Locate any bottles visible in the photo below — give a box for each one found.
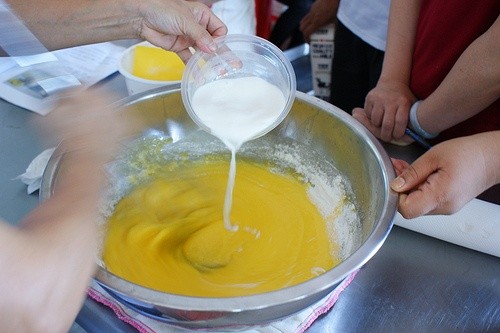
[310,24,335,97]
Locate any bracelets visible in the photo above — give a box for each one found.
[406,100,436,145]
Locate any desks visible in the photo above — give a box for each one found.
[0,61,500,333]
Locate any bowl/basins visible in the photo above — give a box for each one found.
[39,82,400,329]
[116,41,182,96]
[181,33,297,142]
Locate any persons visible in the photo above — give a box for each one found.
[298,0,500,219]
[0,1,245,333]
